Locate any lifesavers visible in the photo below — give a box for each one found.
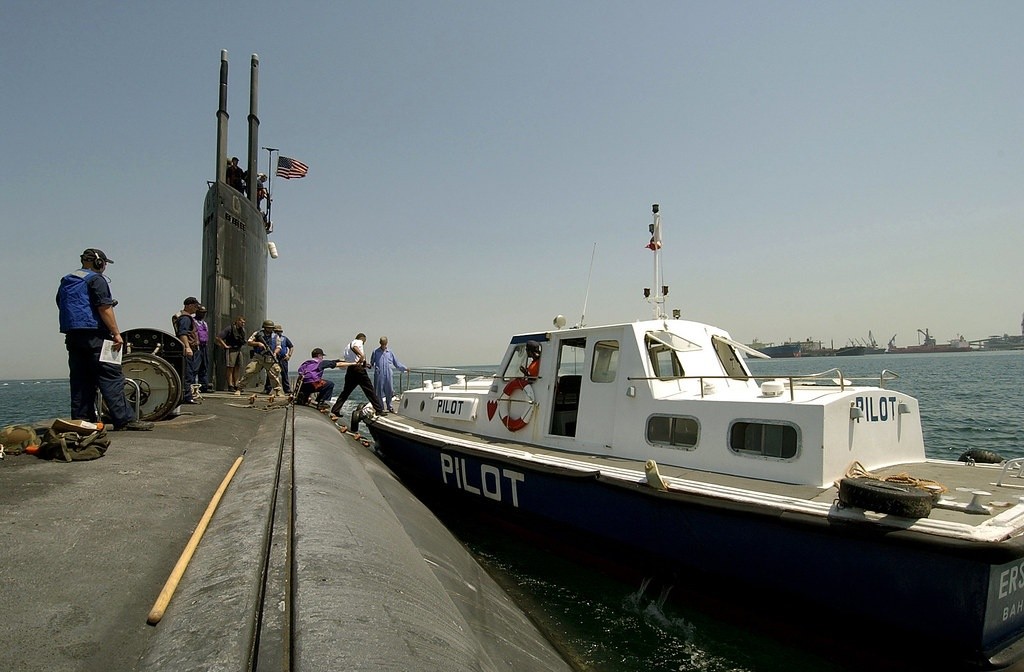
[838,475,933,519]
[498,377,536,433]
[351,410,359,431]
[958,446,1005,464]
[356,401,367,420]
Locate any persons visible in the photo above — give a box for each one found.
[296,349,361,409]
[331,333,388,416]
[258,324,294,396]
[519,341,540,377]
[234,320,284,398]
[227,157,247,195]
[193,305,214,392]
[367,336,408,413]
[244,171,263,184]
[257,175,268,211]
[175,297,201,403]
[56,248,154,429]
[214,316,246,393]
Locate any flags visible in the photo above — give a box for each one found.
[276,156,308,179]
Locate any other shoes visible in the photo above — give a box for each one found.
[317,400,331,410]
[202,388,214,393]
[376,409,387,416]
[184,399,200,405]
[390,407,397,414]
[228,386,236,392]
[285,389,293,394]
[333,411,344,417]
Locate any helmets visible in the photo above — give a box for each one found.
[198,305,206,312]
[262,320,275,328]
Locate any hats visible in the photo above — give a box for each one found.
[273,325,283,331]
[312,349,326,357]
[84,248,113,263]
[527,341,541,359]
[184,296,201,305]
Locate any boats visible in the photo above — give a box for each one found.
[355,202,1024,672]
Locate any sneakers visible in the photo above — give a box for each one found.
[114,418,154,432]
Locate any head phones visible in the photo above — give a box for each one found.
[88,249,104,270]
